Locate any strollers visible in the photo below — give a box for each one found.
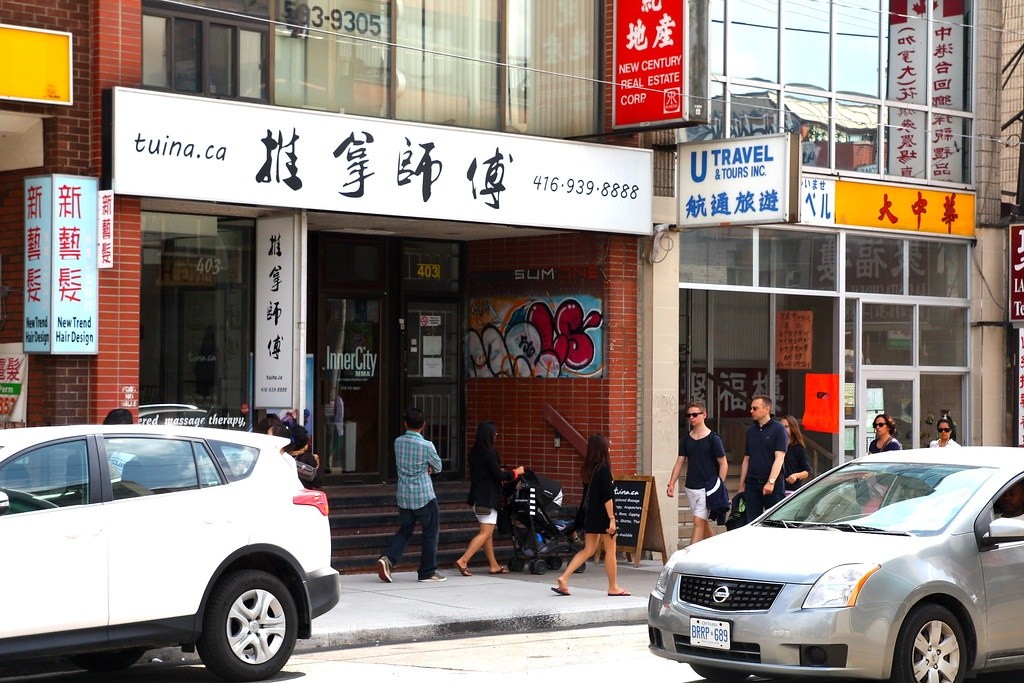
[499,463,587,575]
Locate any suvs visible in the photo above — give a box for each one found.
[0,423,341,683]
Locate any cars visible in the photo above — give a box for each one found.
[645,445,1023,683]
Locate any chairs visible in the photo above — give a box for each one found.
[56,456,84,504]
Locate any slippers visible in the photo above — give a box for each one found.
[608,590,631,596]
[551,587,571,595]
[455,561,472,575]
[490,565,510,574]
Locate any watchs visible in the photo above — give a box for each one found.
[768,479,774,483]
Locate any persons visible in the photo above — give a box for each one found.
[666,401,728,546]
[738,396,789,524]
[101,408,322,515]
[779,415,813,492]
[556,434,632,596]
[377,408,447,583]
[859,414,903,520]
[930,415,961,448]
[455,421,525,576]
[326,386,345,465]
[993,482,1024,520]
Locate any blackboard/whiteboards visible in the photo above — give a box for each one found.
[595,475,668,552]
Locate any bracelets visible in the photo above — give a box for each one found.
[667,483,674,488]
[610,517,615,520]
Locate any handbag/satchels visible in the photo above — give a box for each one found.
[295,460,316,480]
[475,501,492,515]
[573,494,589,529]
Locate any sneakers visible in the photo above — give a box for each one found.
[377,556,393,582]
[419,572,446,582]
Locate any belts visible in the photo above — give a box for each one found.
[752,479,766,484]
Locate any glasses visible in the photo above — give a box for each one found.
[873,422,888,428]
[686,412,704,418]
[937,427,950,433]
[749,406,766,410]
